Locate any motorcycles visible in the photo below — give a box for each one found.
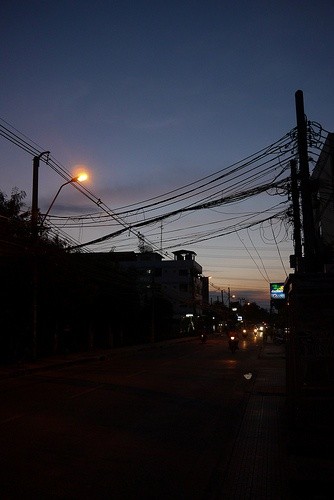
[200,331,208,345]
[227,335,239,353]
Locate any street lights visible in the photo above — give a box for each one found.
[38,178,79,239]
